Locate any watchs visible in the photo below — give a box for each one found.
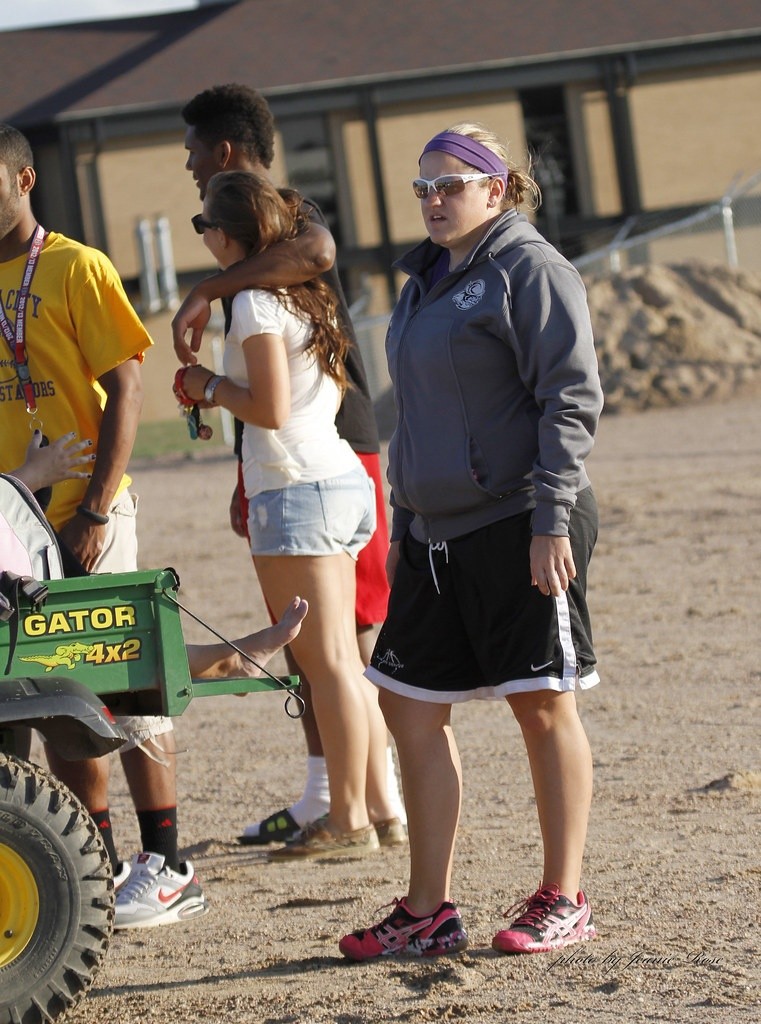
[205,376,227,405]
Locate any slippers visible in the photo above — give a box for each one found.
[237,808,300,844]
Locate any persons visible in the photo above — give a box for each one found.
[0,84,408,930]
[339,122,607,959]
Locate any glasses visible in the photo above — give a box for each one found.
[191,214,229,234]
[412,172,505,198]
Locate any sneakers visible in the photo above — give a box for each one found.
[113,852,210,930]
[339,895,469,961]
[375,817,406,845]
[491,880,596,954]
[114,860,133,889]
[267,814,380,863]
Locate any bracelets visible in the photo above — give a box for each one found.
[76,505,109,524]
[203,373,215,394]
